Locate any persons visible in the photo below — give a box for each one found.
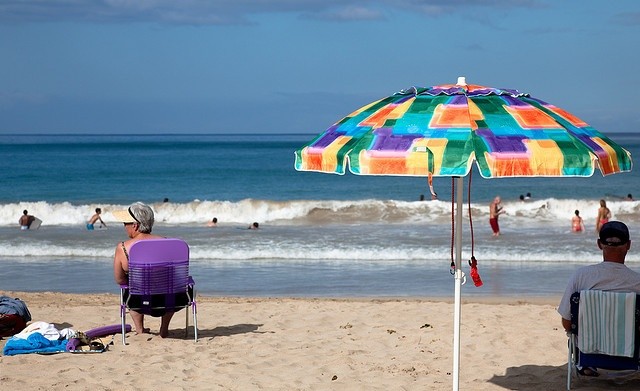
[519,194,524,204]
[19,210,33,229]
[572,210,582,233]
[490,196,504,236]
[595,200,610,232]
[108,201,172,337]
[524,193,531,199]
[87,208,107,230]
[164,198,168,202]
[557,221,640,332]
[253,222,258,230]
[625,193,633,201]
[419,194,425,200]
[208,218,217,227]
[432,192,437,201]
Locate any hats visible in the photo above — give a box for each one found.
[112,206,140,224]
[599,221,630,245]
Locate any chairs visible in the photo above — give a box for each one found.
[119,239,197,346]
[566,289,640,391]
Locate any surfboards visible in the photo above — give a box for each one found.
[94,227,108,230]
[19,216,42,229]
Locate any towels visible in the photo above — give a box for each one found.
[576,290,636,357]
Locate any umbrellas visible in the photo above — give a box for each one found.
[295,77,633,391]
